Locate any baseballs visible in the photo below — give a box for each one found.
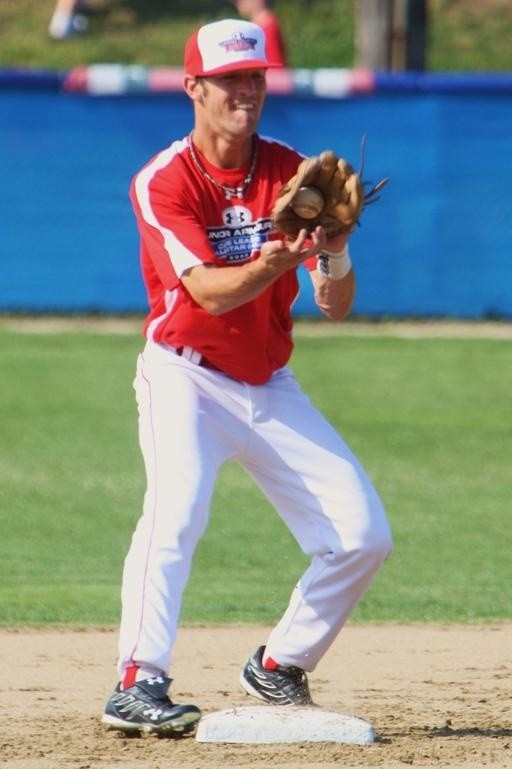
[293,184,323,218]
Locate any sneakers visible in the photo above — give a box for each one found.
[100,674,202,734]
[240,645,313,708]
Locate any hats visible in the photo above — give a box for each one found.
[184,18,285,78]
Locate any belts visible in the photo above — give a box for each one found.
[176,344,225,373]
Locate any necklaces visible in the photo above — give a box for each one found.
[184,129,262,195]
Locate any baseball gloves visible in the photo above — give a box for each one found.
[270,148,389,241]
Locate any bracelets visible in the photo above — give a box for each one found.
[316,239,352,281]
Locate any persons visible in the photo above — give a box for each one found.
[91,11,400,737]
[234,0,288,71]
